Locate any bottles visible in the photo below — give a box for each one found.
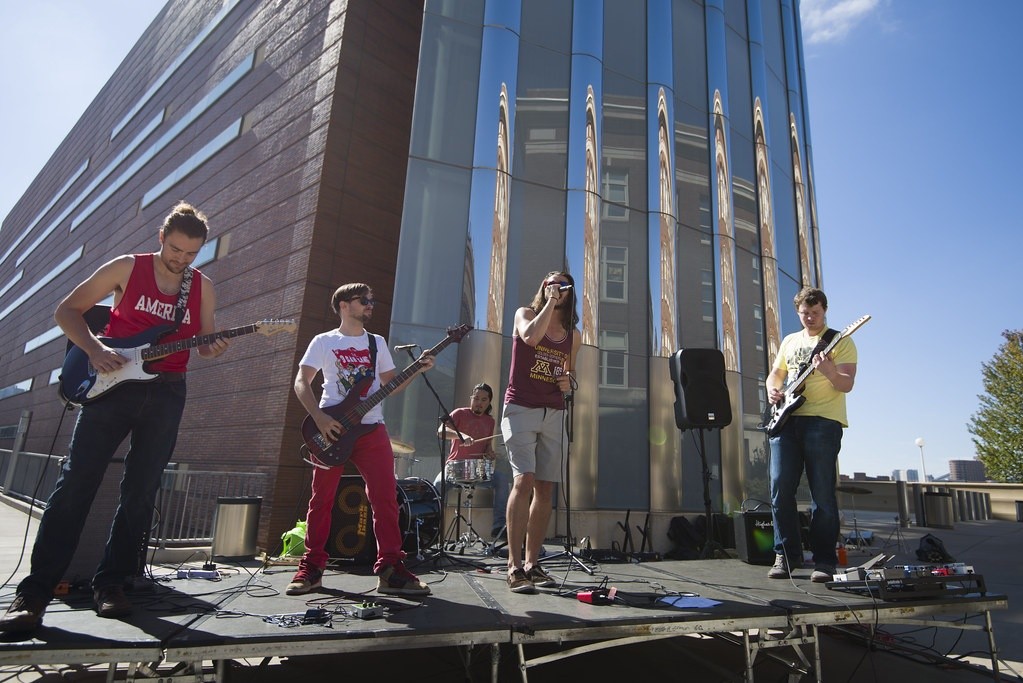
[839,544,848,566]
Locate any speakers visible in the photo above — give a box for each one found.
[668,348,732,431]
[60,430,157,587]
[733,510,805,566]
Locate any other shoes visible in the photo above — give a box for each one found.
[491,527,508,540]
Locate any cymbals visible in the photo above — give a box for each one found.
[836,486,874,496]
[496,442,506,447]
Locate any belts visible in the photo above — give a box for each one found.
[154,372,185,381]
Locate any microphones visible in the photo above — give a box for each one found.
[394,344,418,353]
[558,285,572,293]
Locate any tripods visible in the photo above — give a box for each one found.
[844,492,913,556]
[537,389,596,575]
[405,350,492,574]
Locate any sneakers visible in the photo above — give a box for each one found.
[768,554,801,578]
[507,568,535,592]
[525,566,556,585]
[378,562,430,593]
[0,596,42,630]
[286,559,321,594]
[94,585,134,617]
[811,562,835,581]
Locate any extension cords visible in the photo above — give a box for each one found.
[177,570,217,579]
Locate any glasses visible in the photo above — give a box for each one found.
[548,282,571,287]
[342,296,376,306]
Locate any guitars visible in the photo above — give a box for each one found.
[60,309,298,407]
[300,322,476,467]
[761,313,872,438]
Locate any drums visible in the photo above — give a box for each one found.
[445,457,496,485]
[395,478,444,554]
[390,439,419,480]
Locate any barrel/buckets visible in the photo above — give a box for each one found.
[211,495,262,562]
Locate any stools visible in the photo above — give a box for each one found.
[448,483,489,556]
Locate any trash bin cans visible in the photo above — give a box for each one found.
[210,495,263,562]
[1015,500,1023,522]
[924,492,955,528]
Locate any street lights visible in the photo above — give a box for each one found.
[915,437,927,482]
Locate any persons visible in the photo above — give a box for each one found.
[285,284,436,595]
[434,383,508,541]
[0,204,232,630]
[766,288,859,583]
[501,271,582,594]
